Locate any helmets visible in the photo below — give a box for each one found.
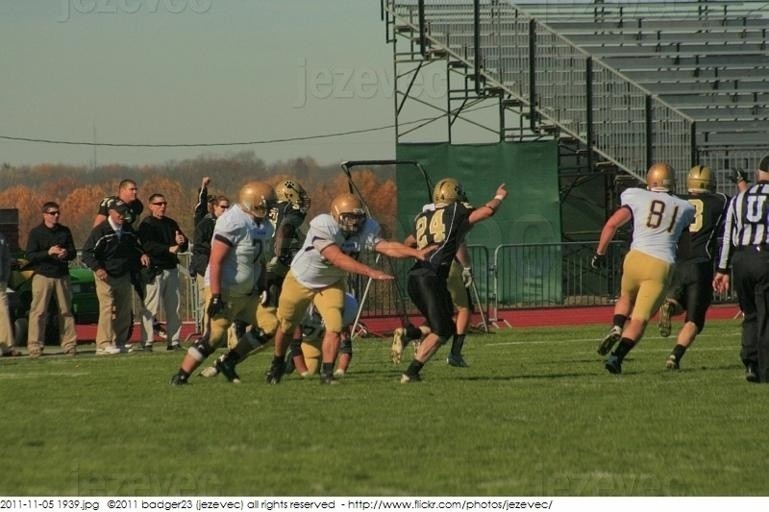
[433,177,464,205]
[647,163,715,193]
[330,194,367,235]
[240,180,310,220]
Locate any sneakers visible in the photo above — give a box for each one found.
[266,350,347,389]
[667,355,680,369]
[169,354,241,384]
[658,303,671,337]
[392,327,469,384]
[598,325,623,374]
[95,344,185,355]
[29,349,42,359]
[4,349,22,356]
[66,347,76,356]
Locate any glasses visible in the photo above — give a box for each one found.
[153,202,166,206]
[47,211,60,215]
[220,205,229,208]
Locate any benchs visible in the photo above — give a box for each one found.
[394,0,769,196]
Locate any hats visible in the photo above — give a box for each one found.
[107,198,128,212]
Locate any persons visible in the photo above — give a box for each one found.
[591,163,696,374]
[0,235,21,356]
[81,198,151,356]
[172,177,507,386]
[137,194,188,352]
[713,157,769,383]
[25,202,76,358]
[92,179,168,349]
[659,163,748,369]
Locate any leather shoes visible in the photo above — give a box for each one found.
[746,364,759,382]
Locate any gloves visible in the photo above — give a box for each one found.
[591,254,606,270]
[725,166,746,183]
[207,293,223,316]
[258,287,269,306]
[461,267,472,287]
